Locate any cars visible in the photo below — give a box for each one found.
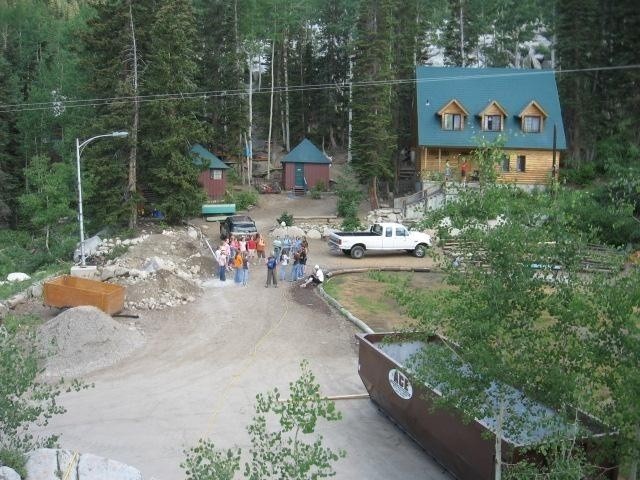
[219,214,258,240]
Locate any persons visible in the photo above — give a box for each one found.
[213,233,309,288]
[460,158,468,185]
[302,264,326,286]
[445,160,454,180]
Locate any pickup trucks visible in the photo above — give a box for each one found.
[330,222,431,258]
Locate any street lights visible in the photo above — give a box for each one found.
[75,131,129,267]
